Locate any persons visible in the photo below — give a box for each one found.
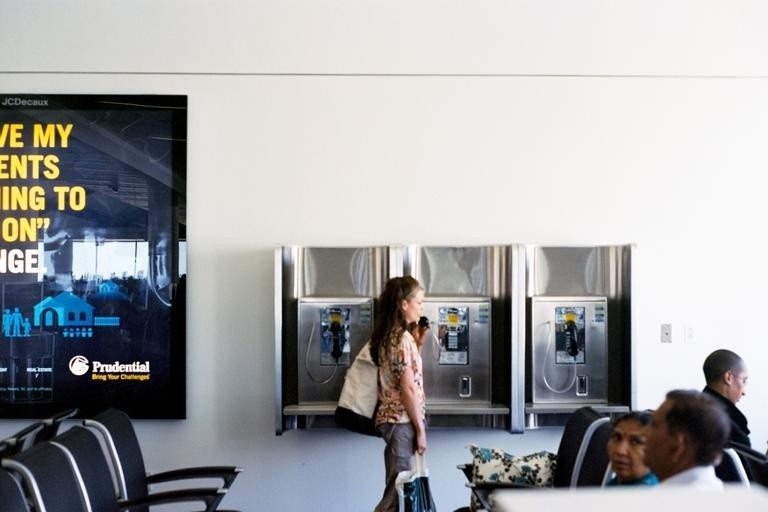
[374,274,432,511]
[602,406,661,485]
[701,349,751,448]
[641,388,731,485]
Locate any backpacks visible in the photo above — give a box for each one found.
[330,342,383,437]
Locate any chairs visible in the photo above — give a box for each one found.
[456,406,768,512]
[0,407,249,512]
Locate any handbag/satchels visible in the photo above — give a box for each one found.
[395,449,437,512]
